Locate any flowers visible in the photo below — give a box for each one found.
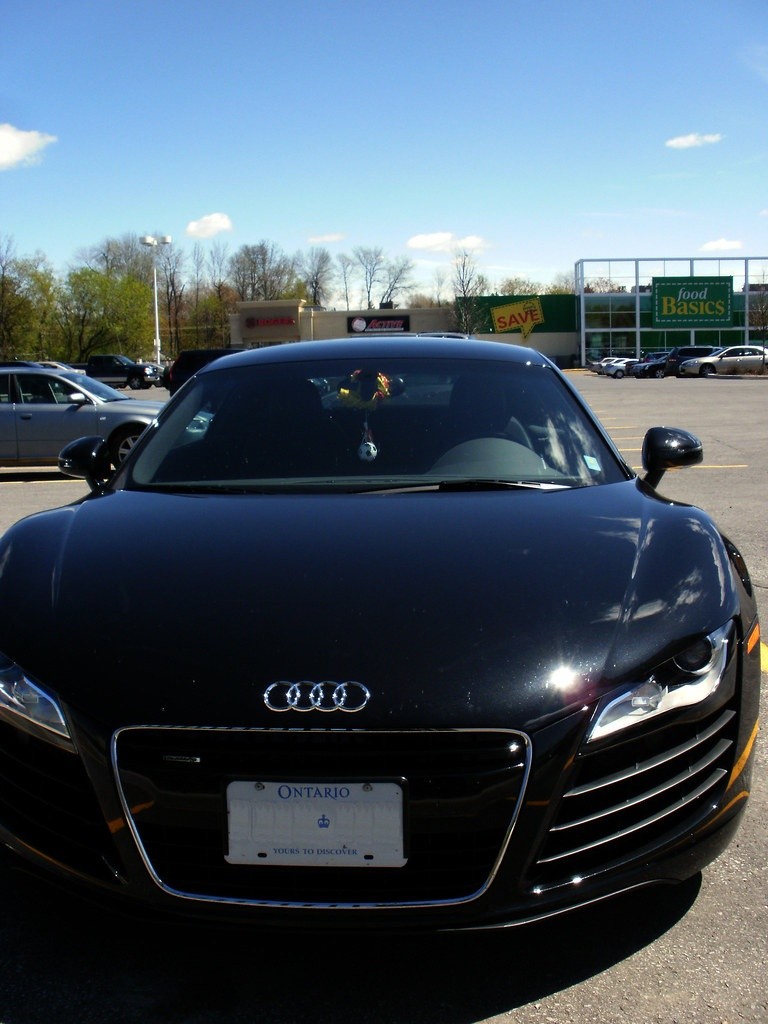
[336,368,393,412]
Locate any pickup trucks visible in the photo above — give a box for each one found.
[67,355,159,390]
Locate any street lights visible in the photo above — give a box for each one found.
[139,234,173,363]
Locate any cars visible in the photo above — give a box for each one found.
[0,336,762,932]
[679,345,768,378]
[588,346,723,379]
[0,361,87,376]
[0,368,214,474]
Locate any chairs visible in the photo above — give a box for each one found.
[418,368,533,469]
[30,384,48,402]
[192,378,339,480]
